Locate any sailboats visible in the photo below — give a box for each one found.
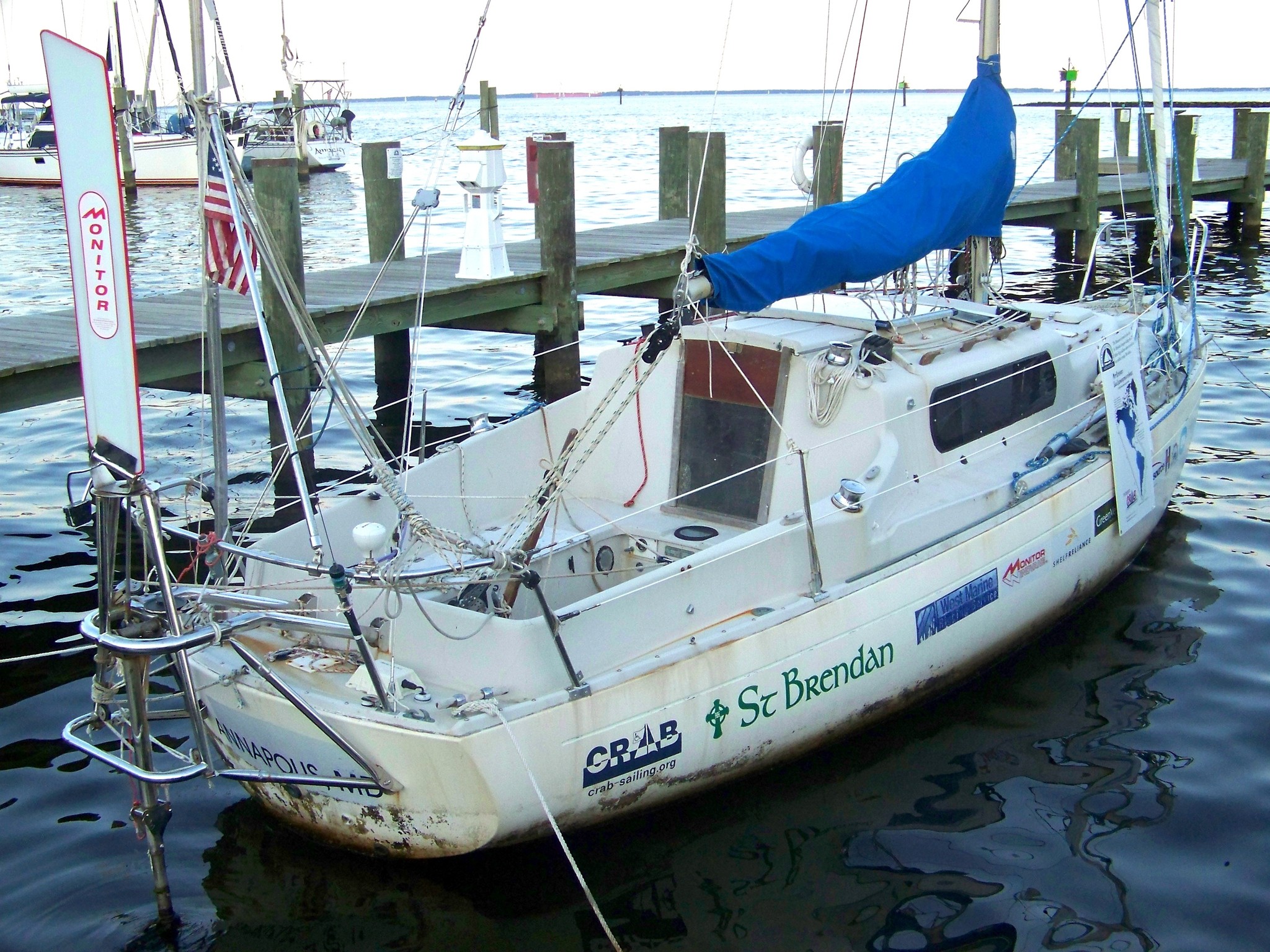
[24,0,1208,862]
[0,0,352,187]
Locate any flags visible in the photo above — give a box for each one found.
[203,128,258,296]
